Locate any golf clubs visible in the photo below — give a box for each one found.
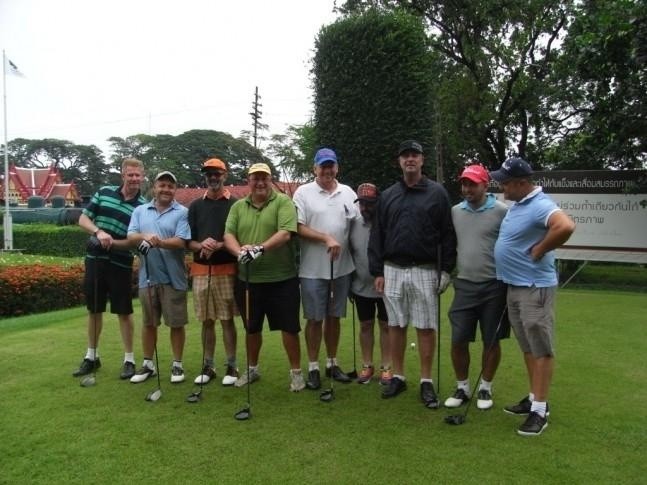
[144,255,163,401]
[234,260,250,418]
[81,246,97,387]
[426,244,441,407]
[447,305,508,422]
[187,260,211,402]
[320,255,334,400]
[346,296,357,378]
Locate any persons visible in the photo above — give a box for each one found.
[71,157,146,380]
[187,158,238,384]
[348,179,393,389]
[224,162,306,394]
[294,147,359,392]
[125,171,190,383]
[443,163,508,409]
[493,158,576,436]
[366,138,458,407]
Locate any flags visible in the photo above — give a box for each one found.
[2,49,29,79]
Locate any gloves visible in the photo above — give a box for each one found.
[136,240,151,256]
[435,271,450,294]
[86,235,101,253]
[238,245,264,265]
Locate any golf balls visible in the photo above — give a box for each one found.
[410,342,415,348]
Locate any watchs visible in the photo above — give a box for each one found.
[94,227,101,234]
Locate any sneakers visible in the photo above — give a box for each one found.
[326,365,350,384]
[235,369,258,386]
[307,370,321,390]
[290,374,305,391]
[194,367,216,384]
[382,378,407,399]
[130,364,157,382]
[72,357,101,376]
[357,365,374,384]
[477,389,493,409]
[420,381,440,408]
[503,395,550,416]
[120,361,135,379]
[222,368,239,385]
[444,388,472,408]
[380,367,391,384]
[518,412,548,436]
[171,366,184,382]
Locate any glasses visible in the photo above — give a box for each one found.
[204,171,225,177]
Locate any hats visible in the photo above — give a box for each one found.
[460,165,488,184]
[490,159,533,183]
[354,183,379,203]
[201,158,226,171]
[248,162,271,176]
[156,171,177,183]
[315,148,337,165]
[399,140,423,154]
